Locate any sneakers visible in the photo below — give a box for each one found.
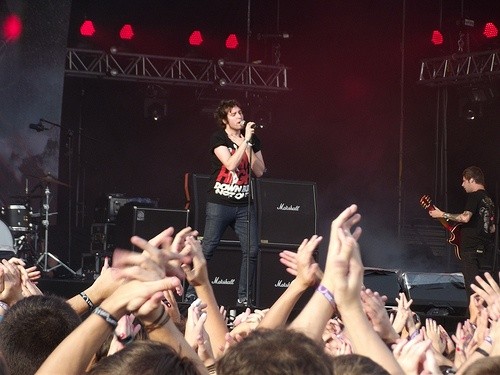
[236,299,256,307]
[185,293,198,304]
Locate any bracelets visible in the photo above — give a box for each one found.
[80,292,93,309]
[485,336,494,344]
[411,330,418,339]
[146,305,170,331]
[93,306,118,331]
[0,302,8,311]
[244,139,248,144]
[317,284,336,310]
[476,348,489,357]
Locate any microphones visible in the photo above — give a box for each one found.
[240,120,263,128]
[29,123,48,130]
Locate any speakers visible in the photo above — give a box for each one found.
[113,174,320,315]
[363,267,468,313]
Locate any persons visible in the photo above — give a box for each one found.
[430,166,500,295]
[199,100,264,308]
[0,204,500,375]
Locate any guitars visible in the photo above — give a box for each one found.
[418,193,462,262]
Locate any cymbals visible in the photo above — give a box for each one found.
[8,193,45,200]
[27,173,73,189]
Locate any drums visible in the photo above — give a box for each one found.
[6,203,30,231]
[0,218,18,257]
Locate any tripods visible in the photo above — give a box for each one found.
[33,184,78,278]
